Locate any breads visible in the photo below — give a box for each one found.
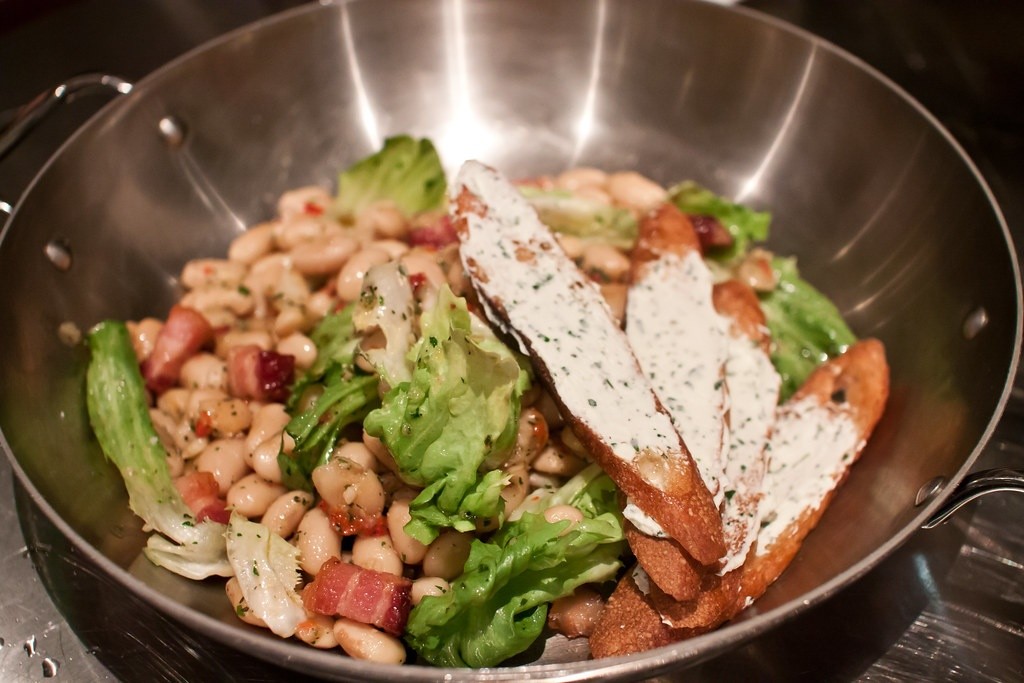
[450,160,891,656]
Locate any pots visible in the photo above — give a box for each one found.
[0,0,1024,683]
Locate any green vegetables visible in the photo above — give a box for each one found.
[85,138,862,668]
